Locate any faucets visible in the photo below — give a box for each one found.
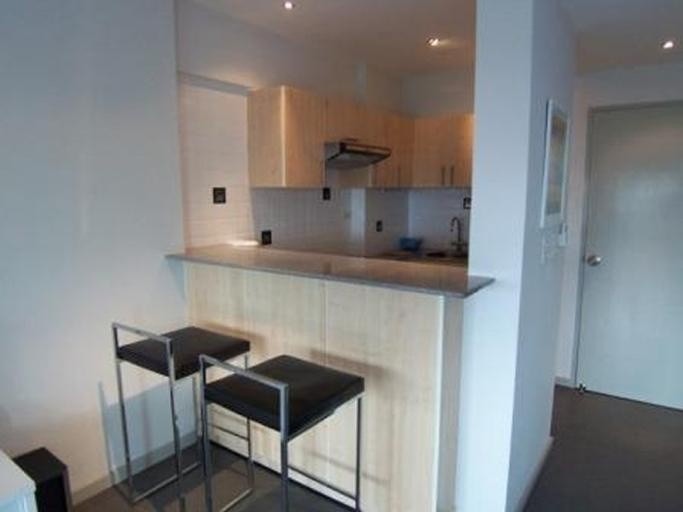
[450,216,468,258]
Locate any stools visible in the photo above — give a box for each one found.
[13,447,68,511]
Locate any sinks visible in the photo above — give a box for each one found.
[426,251,468,259]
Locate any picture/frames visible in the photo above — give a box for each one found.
[540,98,571,230]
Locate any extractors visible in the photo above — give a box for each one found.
[325,142,389,168]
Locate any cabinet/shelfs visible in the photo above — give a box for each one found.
[412,114,474,189]
[366,105,415,188]
[248,85,326,190]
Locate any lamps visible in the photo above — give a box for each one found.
[584,253,604,265]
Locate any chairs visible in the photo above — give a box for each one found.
[111,323,256,512]
[197,353,365,511]
